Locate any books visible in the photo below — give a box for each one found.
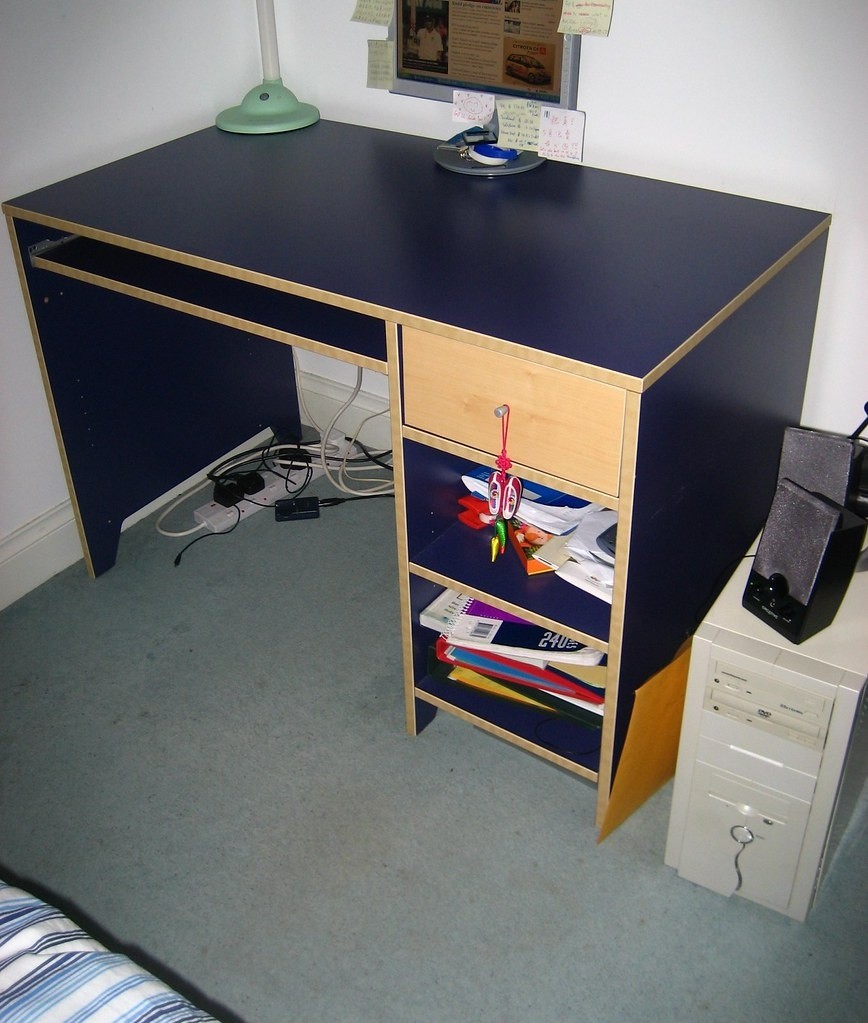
[419,588,608,729]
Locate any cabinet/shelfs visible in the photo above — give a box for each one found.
[0,120,832,831]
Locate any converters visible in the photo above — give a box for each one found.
[273,497,320,522]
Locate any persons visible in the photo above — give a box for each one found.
[416,19,443,65]
[439,23,448,48]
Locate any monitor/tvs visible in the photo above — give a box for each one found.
[385,0,583,174]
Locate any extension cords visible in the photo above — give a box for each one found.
[193,439,357,533]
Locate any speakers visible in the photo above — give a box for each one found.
[740,426,866,646]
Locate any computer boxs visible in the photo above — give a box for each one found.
[661,530,868,922]
